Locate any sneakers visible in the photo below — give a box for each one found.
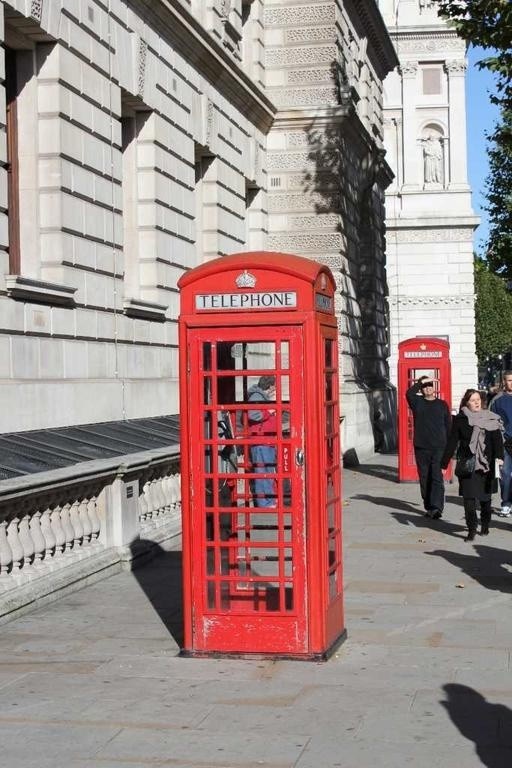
[499,505,511,518]
[480,525,489,536]
[467,528,477,540]
[425,509,443,520]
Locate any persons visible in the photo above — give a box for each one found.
[488,369,511,519]
[405,374,451,522]
[240,373,281,510]
[438,387,505,541]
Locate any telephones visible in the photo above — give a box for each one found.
[217,420,233,461]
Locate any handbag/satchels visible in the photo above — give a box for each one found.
[454,453,475,478]
